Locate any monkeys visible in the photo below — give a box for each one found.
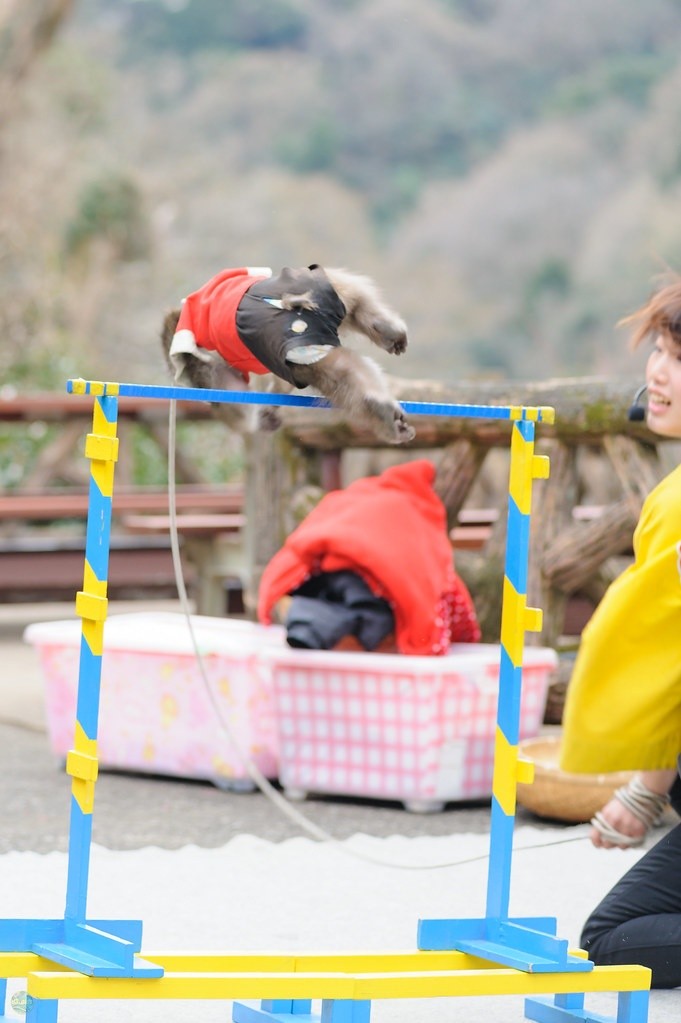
[158,269,417,445]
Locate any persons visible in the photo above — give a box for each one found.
[556,283,681,995]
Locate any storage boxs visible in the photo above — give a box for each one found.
[24,609,295,801]
[264,625,555,817]
